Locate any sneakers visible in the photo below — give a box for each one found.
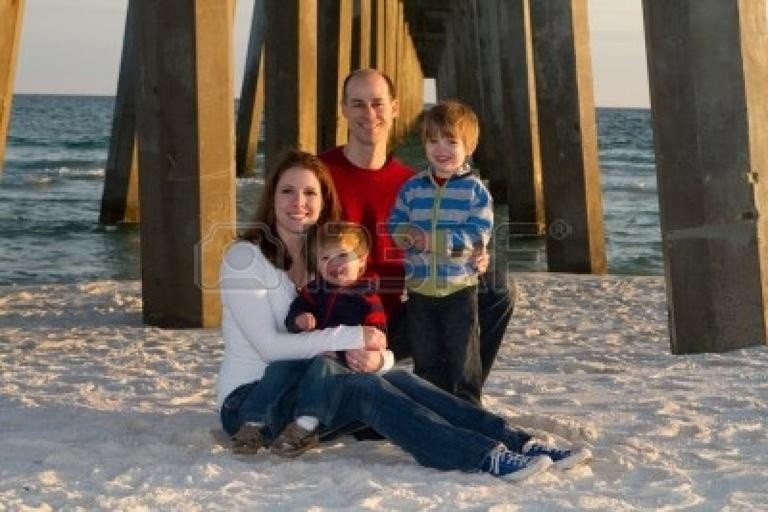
[230,423,269,455]
[482,441,551,483]
[522,437,597,473]
[269,424,323,457]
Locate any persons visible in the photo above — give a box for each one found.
[227,220,388,457]
[386,99,496,414]
[215,148,596,484]
[309,67,520,409]
